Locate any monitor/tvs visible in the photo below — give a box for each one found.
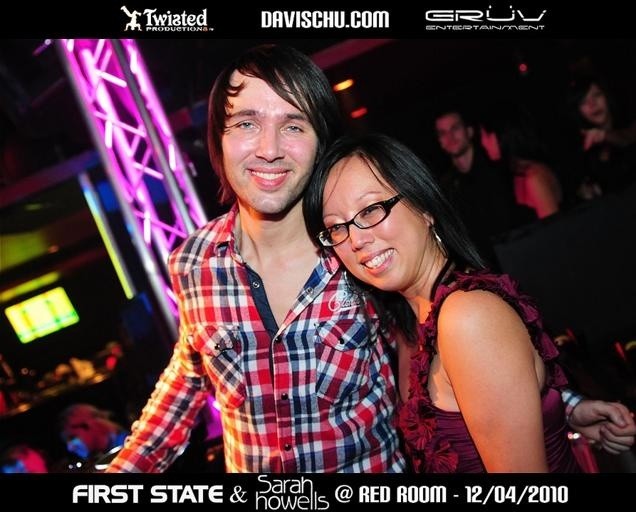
[4,286,82,347]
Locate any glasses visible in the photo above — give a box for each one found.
[319,191,410,247]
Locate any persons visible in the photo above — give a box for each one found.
[101,40,636,474]
[56,405,133,474]
[0,445,49,474]
[299,124,585,478]
[428,67,636,478]
